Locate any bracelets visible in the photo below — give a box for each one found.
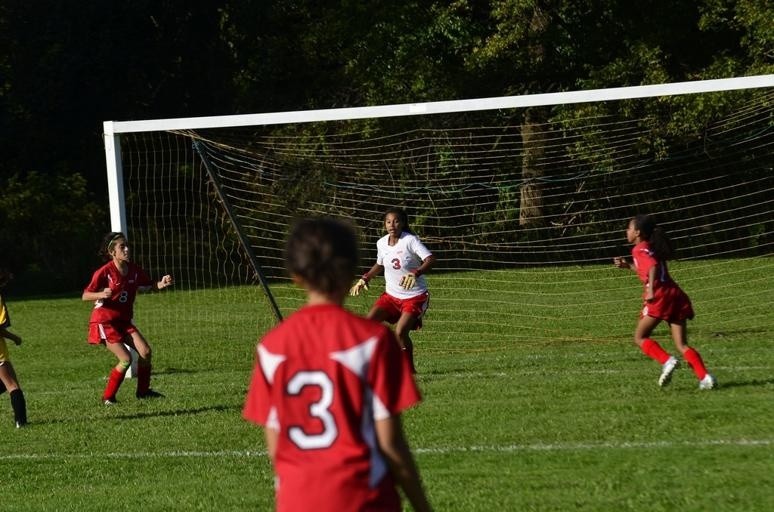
[361,272,370,283]
[411,268,423,278]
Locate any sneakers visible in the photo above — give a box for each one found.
[136,390,165,399]
[657,356,680,387]
[102,396,116,408]
[699,374,719,391]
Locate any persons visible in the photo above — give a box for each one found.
[0,268,35,428]
[610,214,720,391]
[348,205,436,375]
[81,231,176,407]
[240,214,436,512]
